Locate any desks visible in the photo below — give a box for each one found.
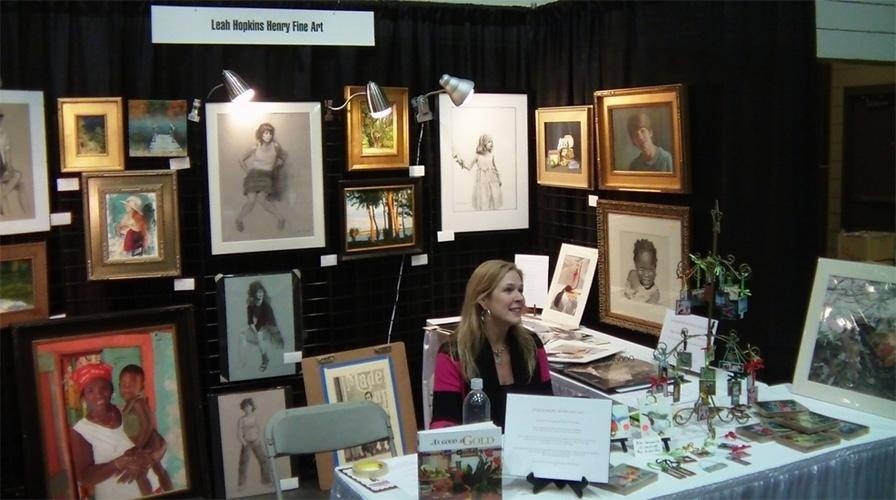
[422,315,769,430]
[330,382,896,500]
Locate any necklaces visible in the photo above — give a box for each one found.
[491,343,509,366]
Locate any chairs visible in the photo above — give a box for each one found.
[265,400,397,500]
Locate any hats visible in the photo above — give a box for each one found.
[120,196,144,217]
[71,362,115,401]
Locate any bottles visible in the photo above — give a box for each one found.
[462,379,491,427]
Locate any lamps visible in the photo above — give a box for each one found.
[416,73,475,124]
[324,82,392,123]
[188,69,255,122]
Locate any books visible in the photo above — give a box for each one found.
[422,315,463,336]
[735,399,871,455]
[521,320,686,396]
[591,463,659,497]
[417,420,503,500]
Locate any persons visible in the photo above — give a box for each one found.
[451,132,506,210]
[117,364,174,496]
[625,112,674,173]
[233,397,273,493]
[233,122,291,232]
[0,113,31,218]
[426,258,557,429]
[623,240,661,304]
[112,196,148,259]
[67,362,168,500]
[238,281,287,372]
[364,391,374,401]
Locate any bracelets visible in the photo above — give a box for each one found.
[114,457,121,473]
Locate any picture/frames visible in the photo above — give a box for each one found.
[216,267,304,382]
[345,86,409,173]
[338,177,424,261]
[206,103,326,257]
[792,256,896,419]
[207,384,299,500]
